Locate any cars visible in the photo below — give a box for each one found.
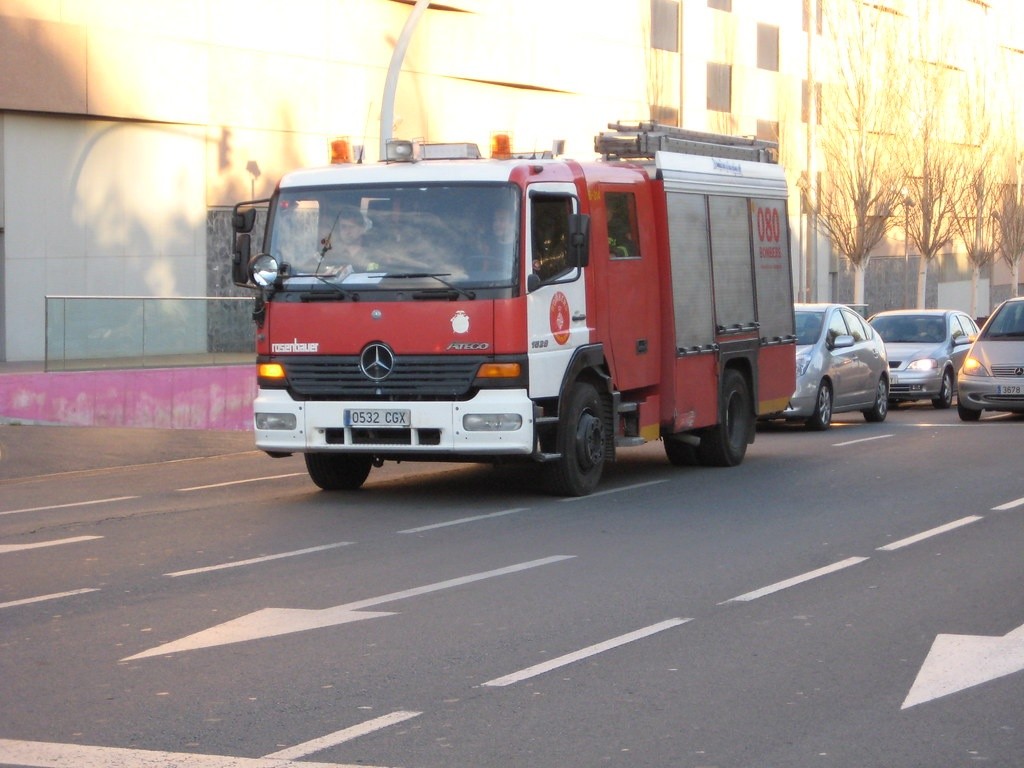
[867,309,983,409]
[768,302,892,431]
[956,296,1024,421]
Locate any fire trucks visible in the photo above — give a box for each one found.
[228,116,802,497]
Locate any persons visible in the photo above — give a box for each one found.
[605,197,635,258]
[804,316,820,336]
[472,203,536,274]
[331,210,383,272]
[881,325,897,337]
[920,321,944,341]
